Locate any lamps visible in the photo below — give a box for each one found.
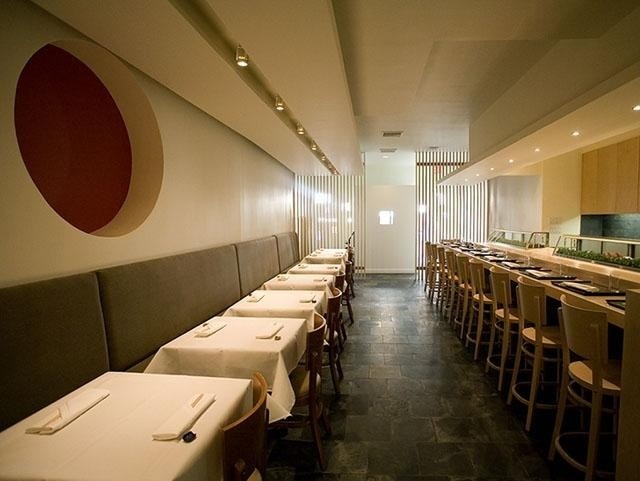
[235,43,249,68]
[312,142,318,151]
[274,95,284,111]
[297,121,304,135]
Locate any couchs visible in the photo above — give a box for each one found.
[273,230,300,273]
[233,235,280,298]
[0,271,111,433]
[95,244,242,375]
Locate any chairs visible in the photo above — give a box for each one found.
[465,255,497,360]
[441,250,460,322]
[272,312,335,460]
[334,270,353,326]
[485,265,534,391]
[436,245,448,309]
[323,287,346,354]
[220,374,268,481]
[453,254,475,336]
[344,261,356,298]
[554,294,615,430]
[424,241,431,291]
[509,277,568,431]
[427,243,440,303]
